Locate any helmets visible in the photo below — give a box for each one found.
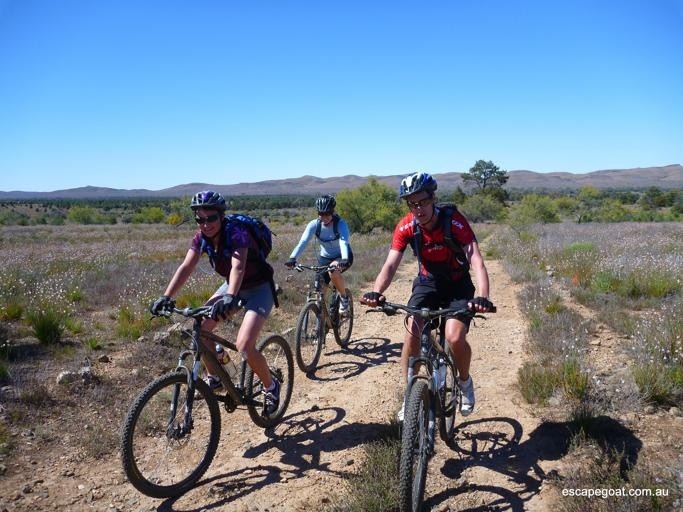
[315,194,336,212]
[190,190,227,211]
[399,171,437,198]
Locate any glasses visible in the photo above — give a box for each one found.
[316,212,331,216]
[406,197,432,209]
[193,213,222,225]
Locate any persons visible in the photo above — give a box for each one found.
[357,167,491,423]
[149,188,279,416]
[282,193,353,340]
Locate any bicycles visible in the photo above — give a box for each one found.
[358,295,497,511]
[120,302,294,498]
[290,262,356,373]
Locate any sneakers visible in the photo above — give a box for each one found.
[459,373,475,416]
[192,376,223,400]
[262,378,281,415]
[338,292,352,318]
[398,395,406,421]
[312,326,329,346]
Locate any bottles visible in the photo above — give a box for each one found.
[214,344,238,379]
[436,358,447,389]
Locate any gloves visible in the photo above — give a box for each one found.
[149,295,170,317]
[283,258,298,270]
[210,294,236,322]
[339,259,349,268]
